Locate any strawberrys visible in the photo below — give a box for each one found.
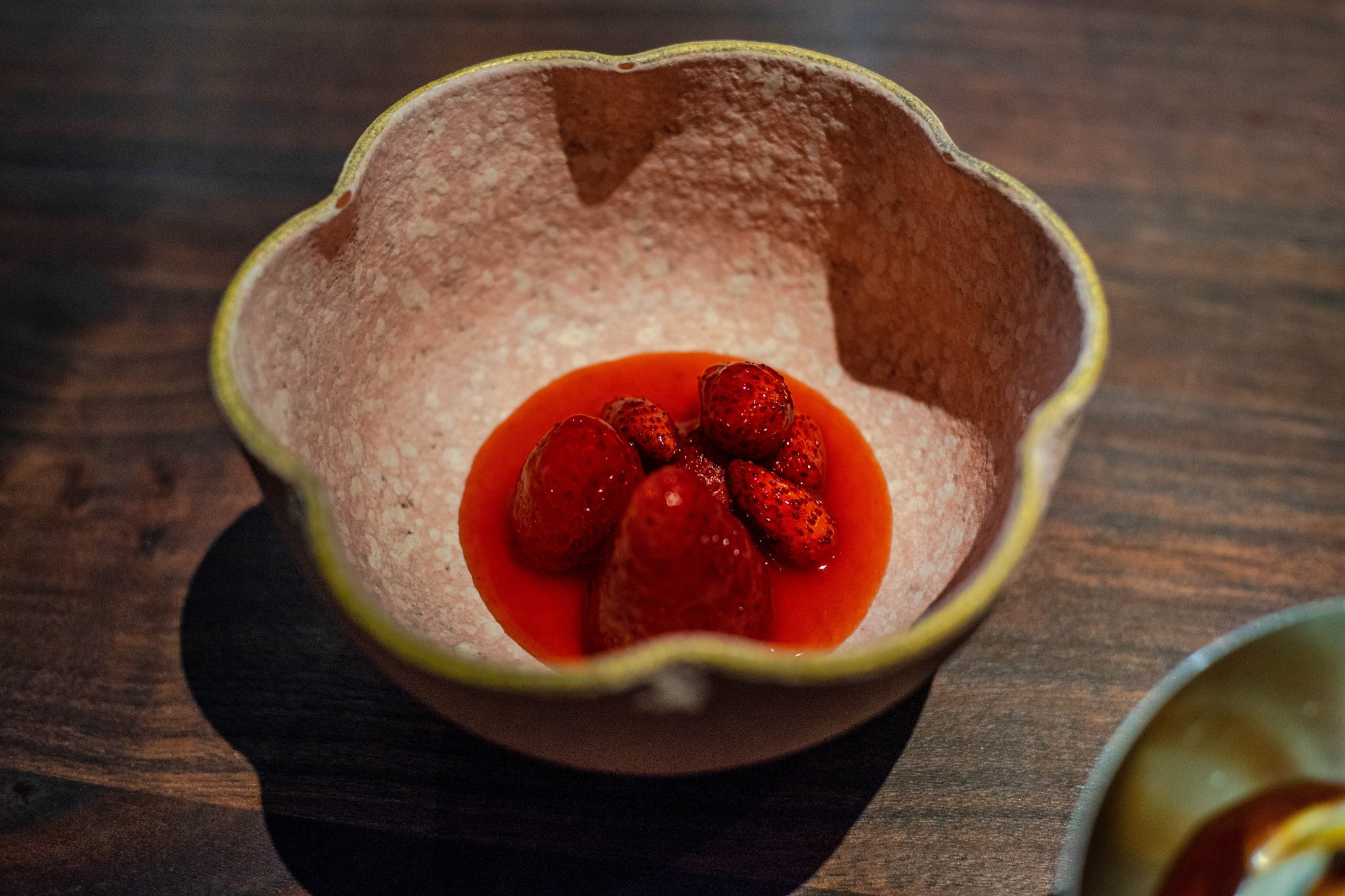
[507,360,836,656]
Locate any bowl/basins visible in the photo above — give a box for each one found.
[1048,595,1345,896]
[206,35,1108,771]
